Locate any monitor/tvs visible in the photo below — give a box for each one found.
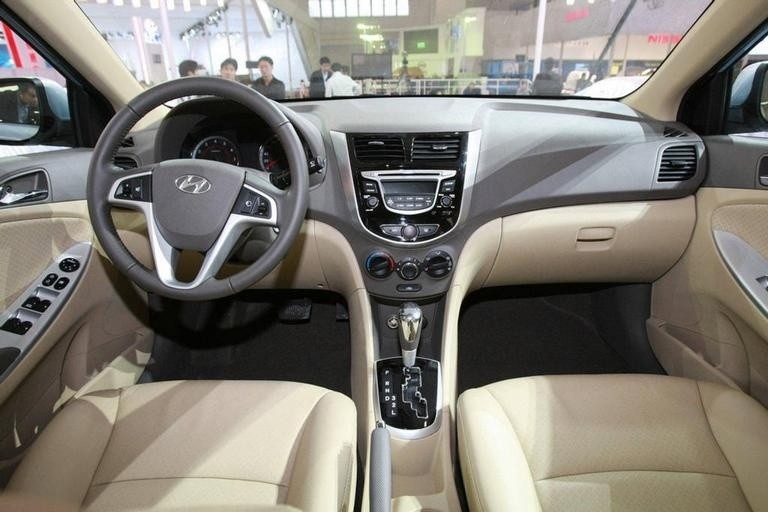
[351,53,392,80]
[403,29,438,54]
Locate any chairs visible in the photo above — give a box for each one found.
[456,373,767,511]
[1,378,358,512]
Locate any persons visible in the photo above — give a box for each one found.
[1,85,38,126]
[575,73,587,93]
[309,56,361,98]
[171,62,199,103]
[531,58,563,97]
[219,56,237,78]
[252,56,286,103]
[463,83,474,95]
